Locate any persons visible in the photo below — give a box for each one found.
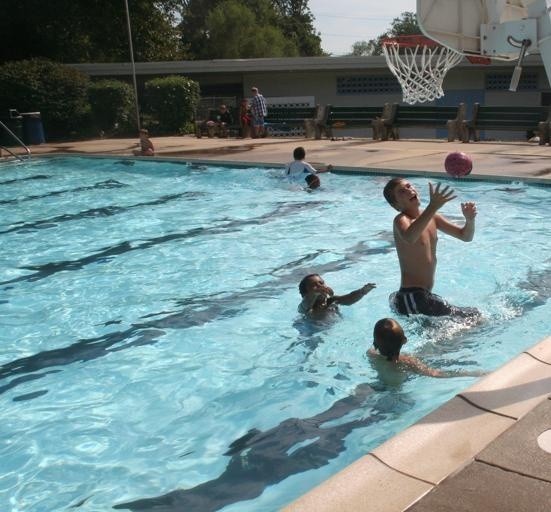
[198,87,267,140]
[296,273,377,320]
[382,178,481,319]
[365,318,485,389]
[131,129,155,157]
[284,147,334,191]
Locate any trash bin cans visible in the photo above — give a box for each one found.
[2,109,47,146]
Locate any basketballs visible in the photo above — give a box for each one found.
[307,176,320,188]
[445,152,473,179]
